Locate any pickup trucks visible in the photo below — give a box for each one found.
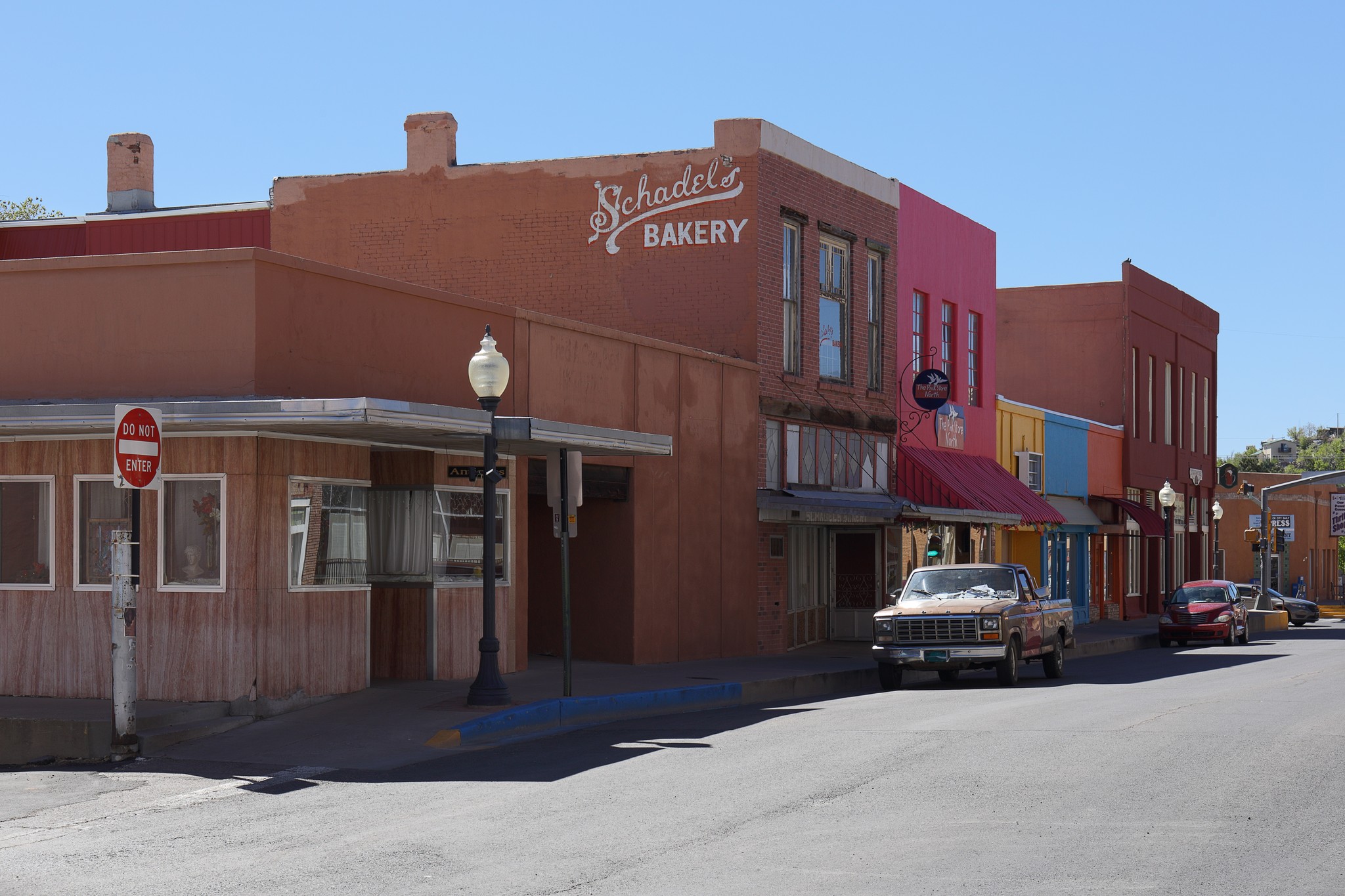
[872,563,1076,684]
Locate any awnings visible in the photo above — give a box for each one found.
[1040,496,1103,525]
[897,445,1068,527]
[1091,494,1175,541]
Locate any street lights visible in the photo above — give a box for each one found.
[1159,476,1176,612]
[1211,498,1224,579]
[467,324,513,706]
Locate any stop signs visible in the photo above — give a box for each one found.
[112,402,163,490]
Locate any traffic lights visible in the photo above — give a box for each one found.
[1243,480,1254,497]
[1273,526,1286,554]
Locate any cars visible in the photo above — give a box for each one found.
[1233,583,1321,627]
[1158,580,1248,649]
